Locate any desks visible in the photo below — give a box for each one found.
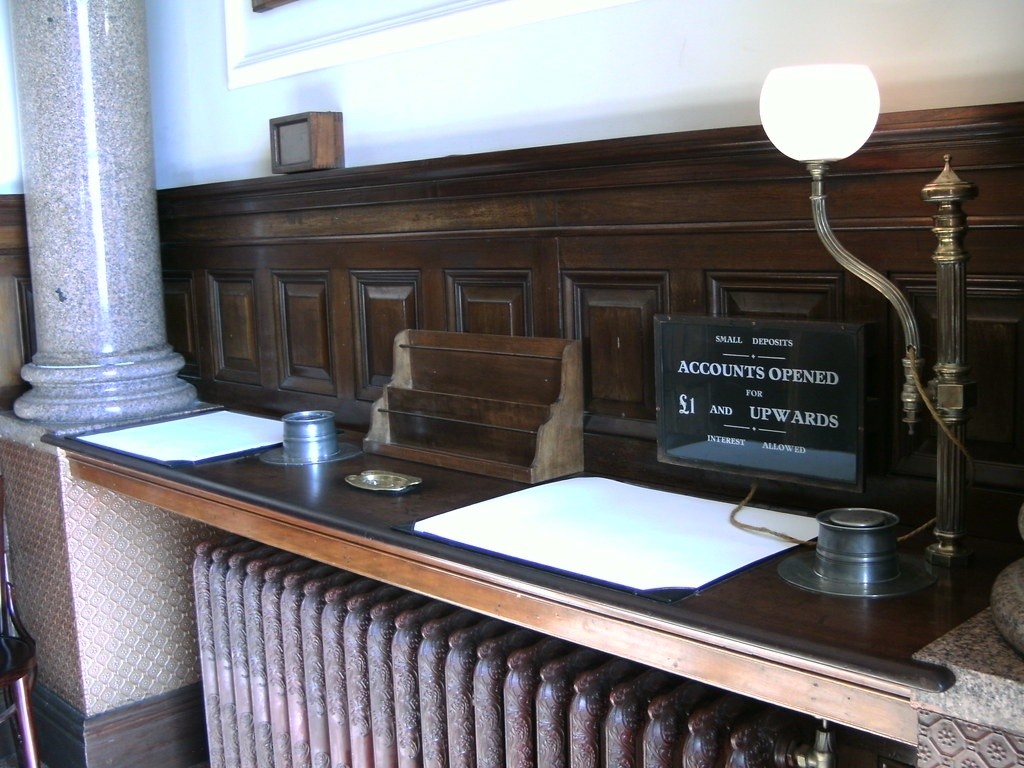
[39,404,997,748]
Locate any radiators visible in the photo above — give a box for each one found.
[192,537,837,768]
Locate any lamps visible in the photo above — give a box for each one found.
[760,60,976,569]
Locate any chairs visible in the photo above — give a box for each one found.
[0,471,43,768]
